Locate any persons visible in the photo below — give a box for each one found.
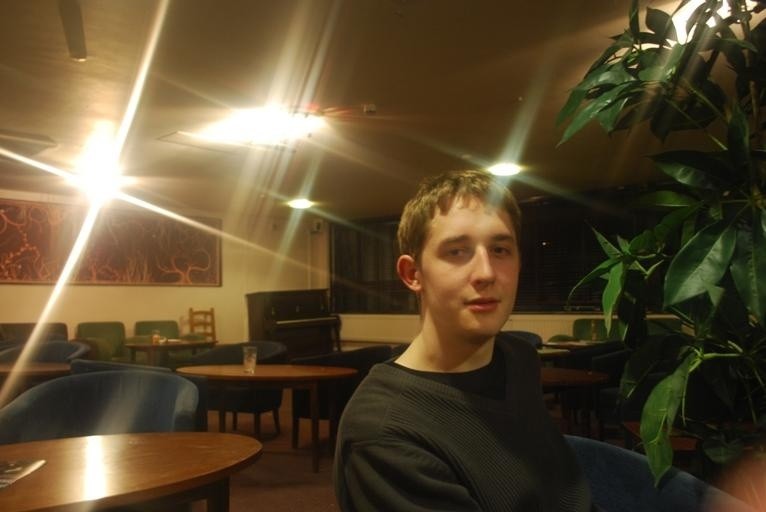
[335,169,606,512]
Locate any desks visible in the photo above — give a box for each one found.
[1,432,263,511]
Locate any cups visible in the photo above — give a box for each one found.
[151,330,161,344]
[241,346,259,374]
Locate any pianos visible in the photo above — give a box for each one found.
[245,287,342,359]
